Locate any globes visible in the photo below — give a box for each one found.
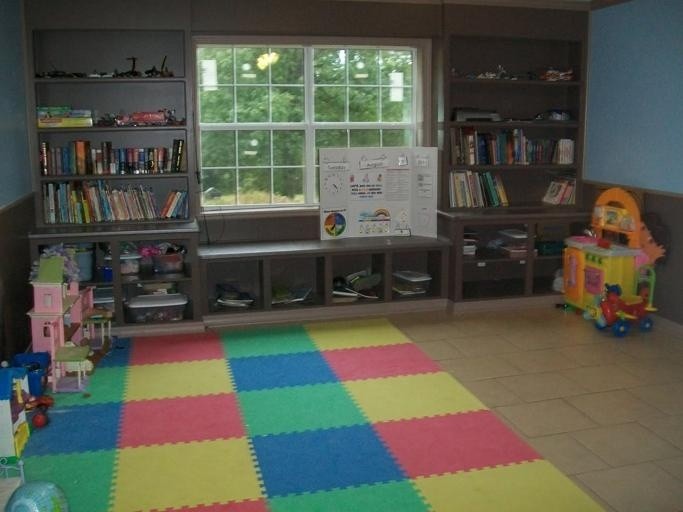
[4,481,74,512]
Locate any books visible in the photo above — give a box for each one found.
[445,128,577,258]
[37,135,186,220]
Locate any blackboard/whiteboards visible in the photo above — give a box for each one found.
[319,147,437,240]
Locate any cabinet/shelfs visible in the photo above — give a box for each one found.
[439,3,593,313]
[18,24,202,341]
[199,234,451,324]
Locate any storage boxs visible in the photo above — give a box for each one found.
[103,253,184,274]
[127,291,188,322]
[392,271,433,296]
[496,227,539,257]
